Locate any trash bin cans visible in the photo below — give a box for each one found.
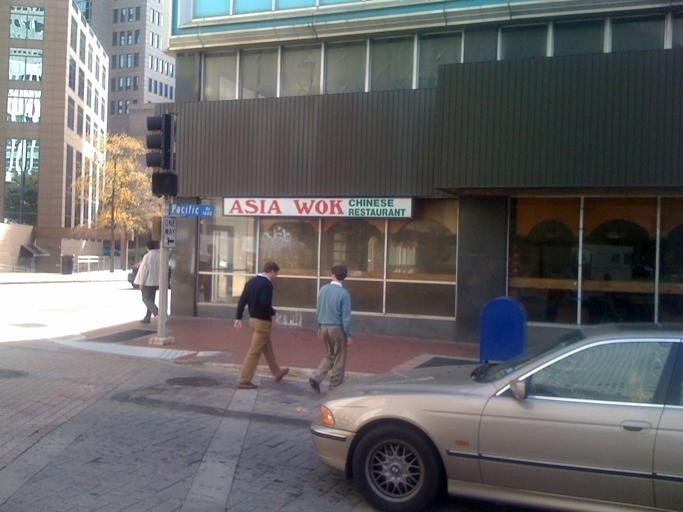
[62,255,74,274]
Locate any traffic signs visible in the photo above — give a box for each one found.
[170,202,214,217]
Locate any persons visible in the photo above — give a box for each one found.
[197,243,218,303]
[132,240,160,323]
[231,262,289,390]
[308,265,353,394]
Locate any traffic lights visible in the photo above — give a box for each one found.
[144,113,172,169]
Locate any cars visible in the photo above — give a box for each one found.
[128,259,175,290]
[311,323,683,510]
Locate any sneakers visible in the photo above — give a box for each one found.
[275,369,288,383]
[309,379,320,394]
[237,382,257,388]
[141,321,150,323]
